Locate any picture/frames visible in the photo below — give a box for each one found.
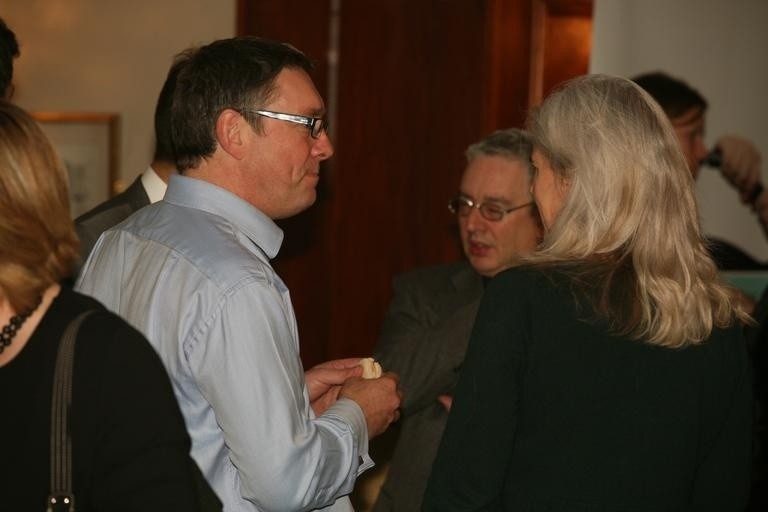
[29,111,122,216]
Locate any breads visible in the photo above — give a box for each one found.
[357,357,382,379]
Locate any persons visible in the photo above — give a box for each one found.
[2,16,32,108]
[71,34,405,512]
[627,70,768,272]
[415,67,768,511]
[351,126,576,511]
[62,44,206,282]
[0,102,227,512]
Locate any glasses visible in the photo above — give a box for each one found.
[236,104,330,139]
[447,197,535,224]
